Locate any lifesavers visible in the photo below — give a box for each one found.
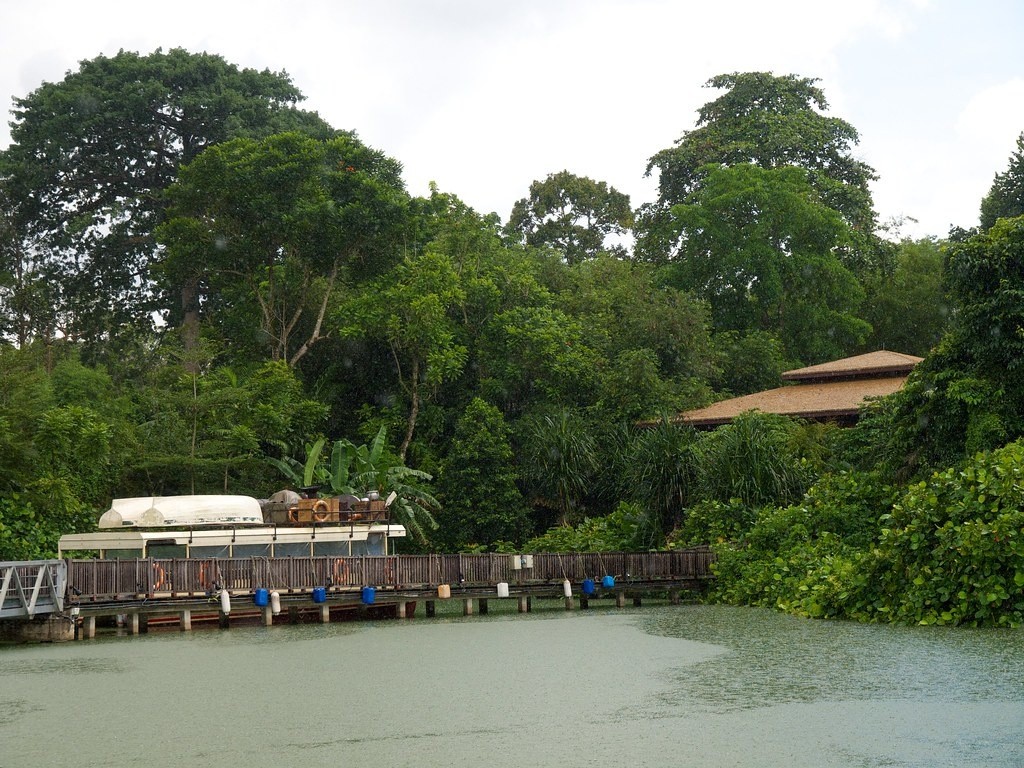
[199,561,220,589]
[312,500,330,522]
[152,562,165,590]
[348,513,362,522]
[332,559,350,581]
[288,506,306,526]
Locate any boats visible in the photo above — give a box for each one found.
[57,495,417,637]
